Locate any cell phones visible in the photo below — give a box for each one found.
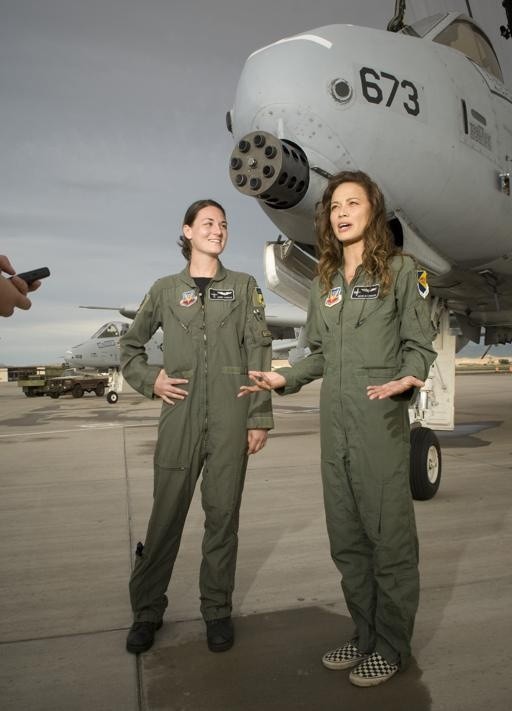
[8,263,51,284]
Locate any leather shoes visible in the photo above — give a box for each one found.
[126,622,164,653]
[205,615,234,651]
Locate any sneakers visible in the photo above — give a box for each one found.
[323,643,369,670]
[349,648,401,688]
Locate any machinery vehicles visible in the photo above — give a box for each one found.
[18,368,109,399]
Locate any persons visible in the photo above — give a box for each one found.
[119,198,272,653]
[238,171,438,688]
[0,255,41,317]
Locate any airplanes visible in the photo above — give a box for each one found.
[120,0,512,500]
[64,321,312,403]
